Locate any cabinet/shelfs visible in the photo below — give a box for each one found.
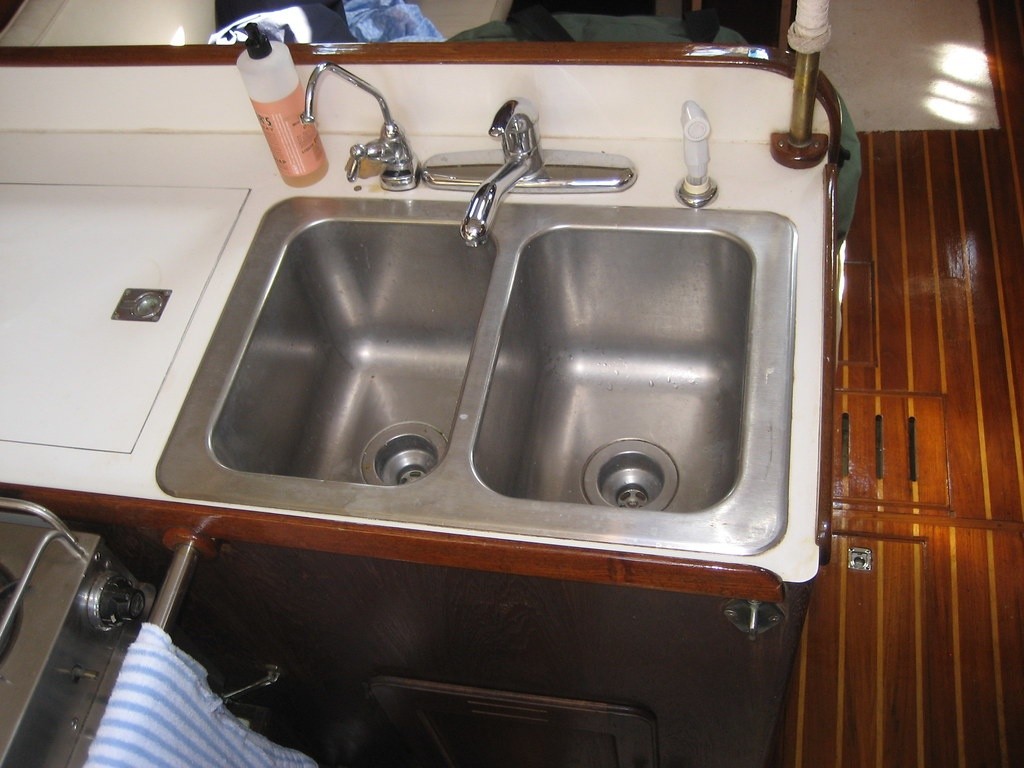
[95,526,817,767]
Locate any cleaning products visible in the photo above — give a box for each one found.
[236,22,329,188]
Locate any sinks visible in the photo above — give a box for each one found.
[470,219,761,521]
[204,213,505,495]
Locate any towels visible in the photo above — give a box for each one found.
[80,621,319,768]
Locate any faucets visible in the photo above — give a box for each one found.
[422,96,639,249]
[300,59,422,192]
